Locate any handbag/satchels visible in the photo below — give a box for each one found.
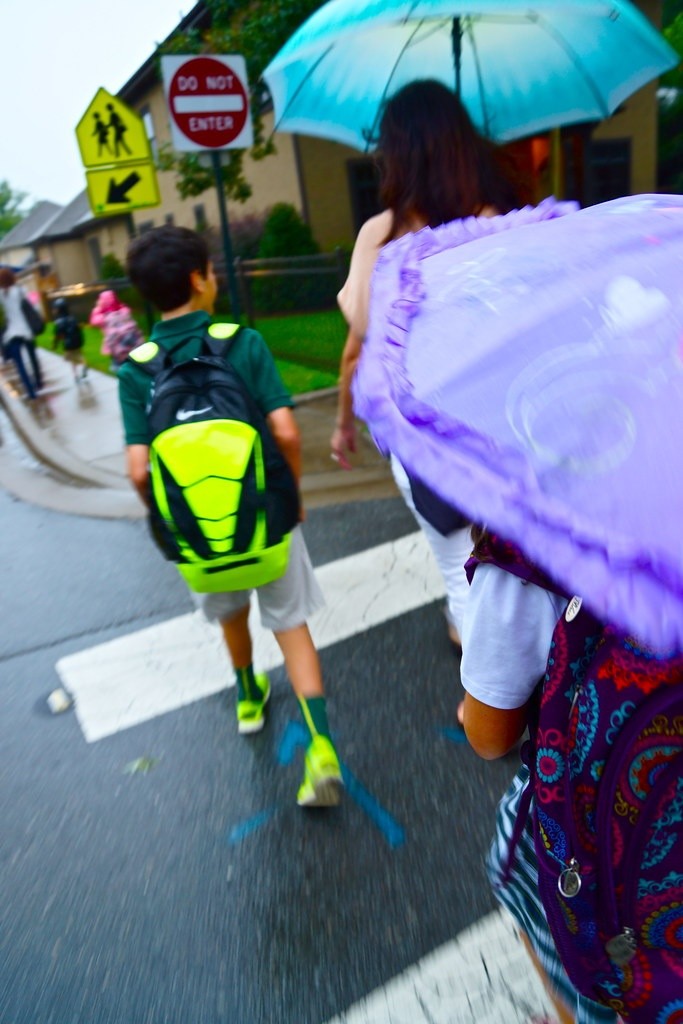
[21,299,44,336]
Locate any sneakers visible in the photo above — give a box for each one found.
[237,672,270,733]
[296,735,344,807]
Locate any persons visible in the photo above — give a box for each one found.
[0,265,144,402]
[331,79,527,647]
[457,526,682,1024]
[116,225,347,808]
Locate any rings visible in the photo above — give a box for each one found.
[330,451,343,461]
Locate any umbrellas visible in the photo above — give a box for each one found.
[351,194,682,660]
[259,0,680,157]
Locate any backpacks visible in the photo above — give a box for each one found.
[465,530,683,1024]
[128,321,294,593]
